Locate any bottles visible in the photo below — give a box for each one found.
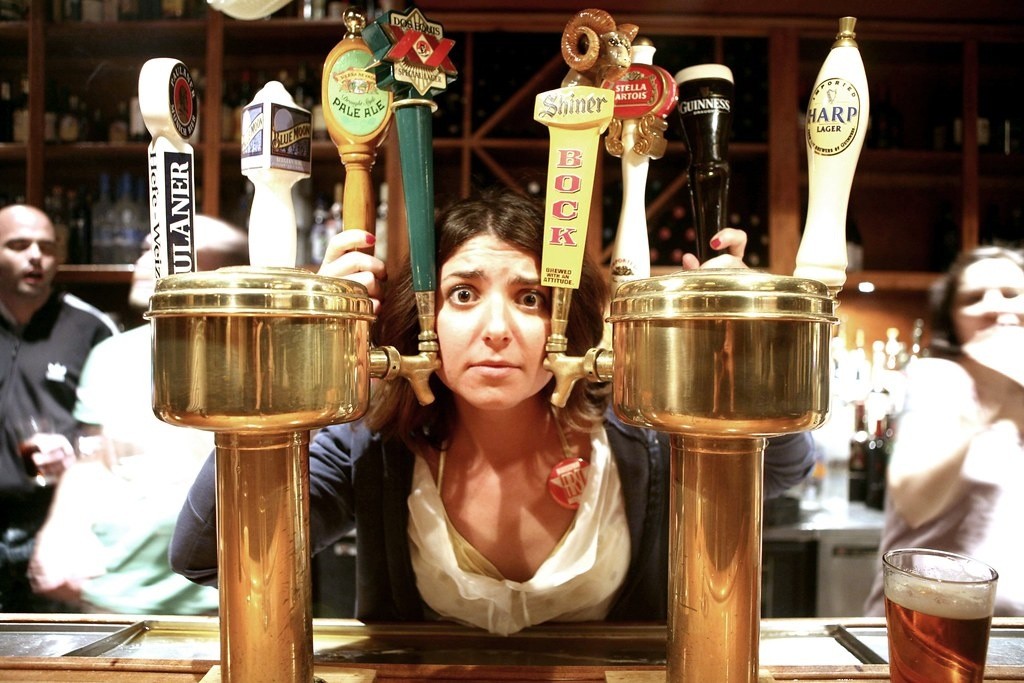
[672,62,735,273]
[950,56,1024,176]
[1,0,356,267]
[789,15,866,288]
[847,402,896,508]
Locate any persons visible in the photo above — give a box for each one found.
[0,205,248,614]
[861,245,1024,615]
[174,191,820,624]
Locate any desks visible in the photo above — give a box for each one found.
[764,503,885,618]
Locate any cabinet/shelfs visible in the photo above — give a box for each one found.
[1,0,1024,358]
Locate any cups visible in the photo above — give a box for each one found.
[882,548,999,683]
